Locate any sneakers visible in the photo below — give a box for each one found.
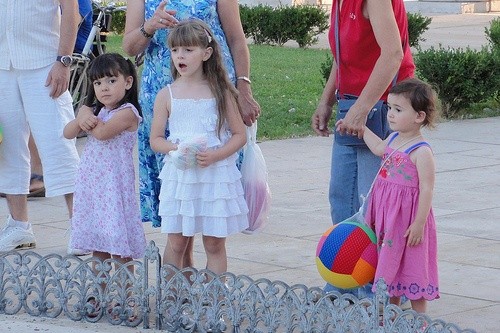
[0,213,37,254]
[63,218,91,256]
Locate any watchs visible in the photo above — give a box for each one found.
[237,76,251,85]
[56,55,72,67]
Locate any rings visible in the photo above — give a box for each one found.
[256,113,259,120]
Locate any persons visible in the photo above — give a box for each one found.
[1,0,89,256]
[149,20,248,329]
[123,1,260,305]
[311,0,416,312]
[0,131,46,197]
[59,0,93,57]
[63,53,145,319]
[336,78,434,331]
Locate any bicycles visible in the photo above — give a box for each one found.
[68,0,147,118]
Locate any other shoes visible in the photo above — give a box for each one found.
[107,303,137,321]
[85,298,99,316]
[204,308,224,332]
[164,307,195,331]
[27,175,45,197]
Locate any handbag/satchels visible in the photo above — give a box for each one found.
[333,93,390,146]
[239,118,272,234]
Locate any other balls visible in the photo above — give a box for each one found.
[316,221,380,288]
